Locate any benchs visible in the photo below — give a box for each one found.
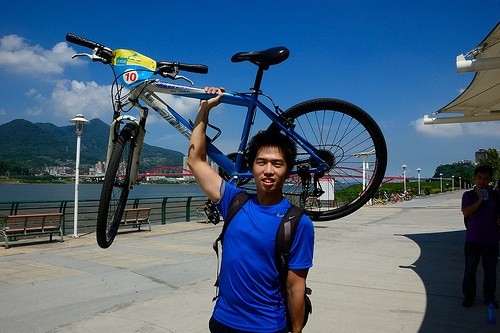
[107,208,152,233]
[0,213,64,249]
[196,200,221,225]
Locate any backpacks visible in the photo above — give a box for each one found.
[213,191,312,333]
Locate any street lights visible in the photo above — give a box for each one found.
[464,182,466,188]
[68,112,91,239]
[451,175,454,189]
[439,173,443,192]
[416,167,421,194]
[459,176,461,190]
[401,164,407,193]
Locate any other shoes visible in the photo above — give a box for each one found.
[484,297,500,309]
[463,298,475,308]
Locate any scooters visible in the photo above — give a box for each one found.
[372,189,412,206]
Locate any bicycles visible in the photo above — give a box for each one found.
[65,32,389,250]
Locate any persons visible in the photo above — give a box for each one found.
[461,164,500,309]
[185,87,315,333]
